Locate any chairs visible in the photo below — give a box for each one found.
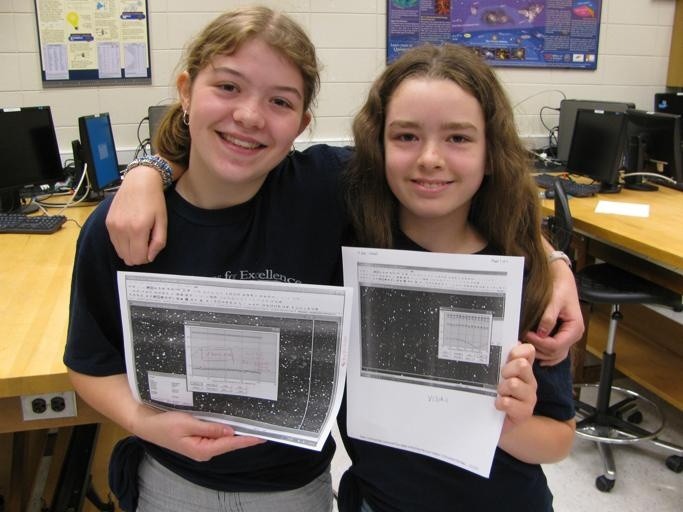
[549,180,683,492]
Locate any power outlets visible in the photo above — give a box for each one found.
[22,391,79,423]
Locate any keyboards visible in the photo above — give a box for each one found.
[0,214,67,234]
[533,174,601,197]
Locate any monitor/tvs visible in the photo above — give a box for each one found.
[72,112,121,202]
[567,109,631,193]
[624,109,683,191]
[0,106,66,214]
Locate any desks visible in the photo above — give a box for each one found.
[2,187,117,512]
[527,161,683,412]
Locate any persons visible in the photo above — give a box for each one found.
[63,9,587,511]
[102,43,577,511]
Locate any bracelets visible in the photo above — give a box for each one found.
[542,249,572,270]
[120,154,175,188]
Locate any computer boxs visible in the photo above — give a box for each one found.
[558,100,635,170]
[149,106,172,156]
[655,93,683,115]
